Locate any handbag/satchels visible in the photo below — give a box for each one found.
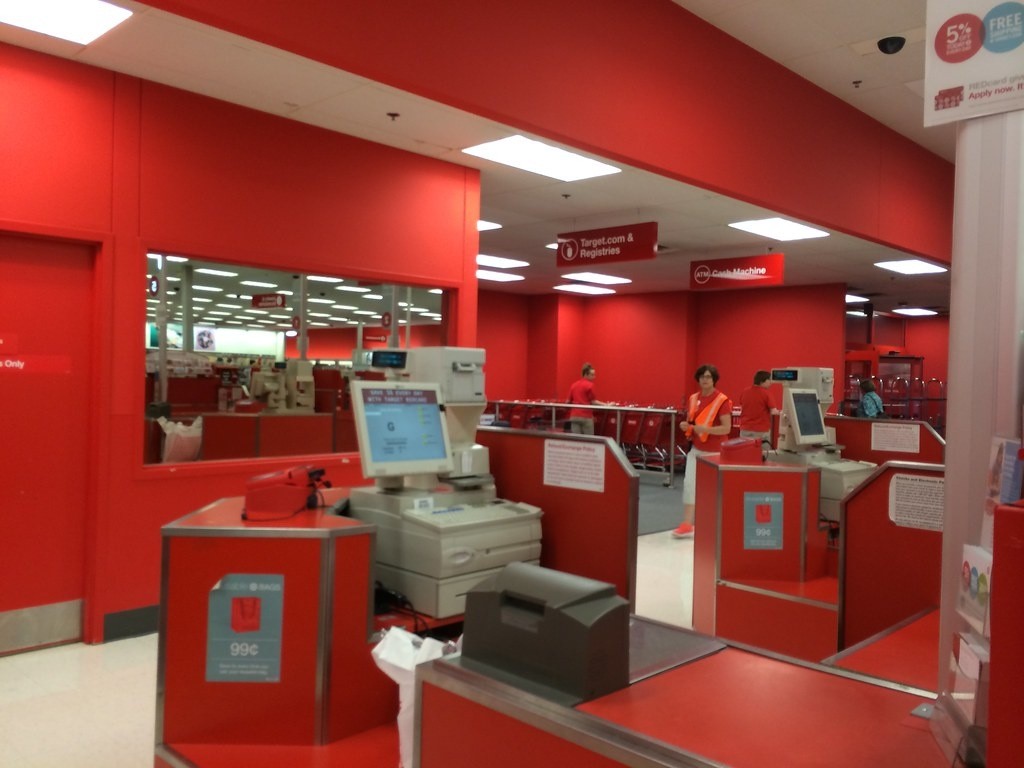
[866,410,883,419]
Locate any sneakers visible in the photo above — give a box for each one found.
[672,521,693,536]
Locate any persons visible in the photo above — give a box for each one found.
[856,380,884,419]
[566,364,617,436]
[738,369,782,452]
[672,364,732,538]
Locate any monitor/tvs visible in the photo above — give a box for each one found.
[351,381,454,496]
[786,388,827,446]
[250,372,284,401]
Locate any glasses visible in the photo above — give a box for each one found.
[699,375,712,379]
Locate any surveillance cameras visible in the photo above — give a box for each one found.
[878,37,906,54]
